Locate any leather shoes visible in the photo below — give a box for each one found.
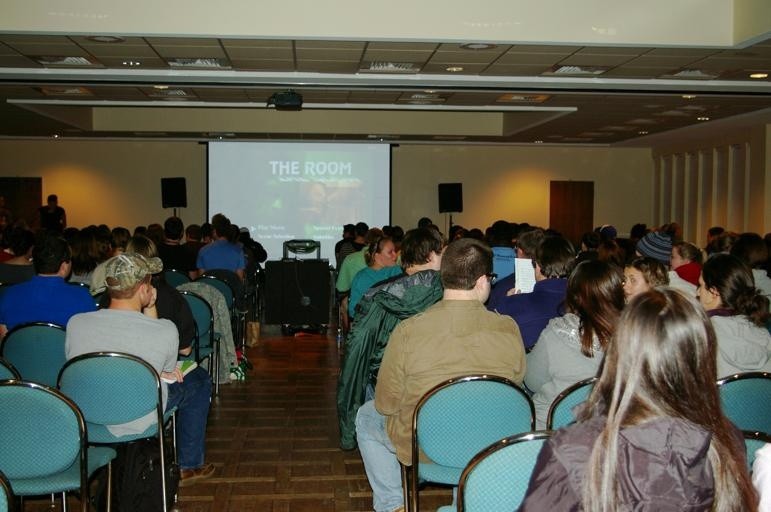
[179,462,215,480]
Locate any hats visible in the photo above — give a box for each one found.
[104,252,164,290]
[635,231,672,261]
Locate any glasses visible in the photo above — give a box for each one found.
[486,274,497,285]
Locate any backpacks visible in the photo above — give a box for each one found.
[88,437,179,512]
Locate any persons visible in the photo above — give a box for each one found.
[29,194,67,234]
[353,237,526,511]
[518,286,760,512]
[524,258,625,431]
[696,254,770,381]
[332,217,770,450]
[1,210,266,386]
[65,254,215,486]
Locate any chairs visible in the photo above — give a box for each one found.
[403,371,771,512]
[0,269,245,512]
[283,239,321,260]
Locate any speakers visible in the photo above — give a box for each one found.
[161,177,187,209]
[438,183,463,213]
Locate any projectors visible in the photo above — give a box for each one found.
[275,92,304,111]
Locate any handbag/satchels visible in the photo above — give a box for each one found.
[245,322,261,348]
[229,354,248,381]
